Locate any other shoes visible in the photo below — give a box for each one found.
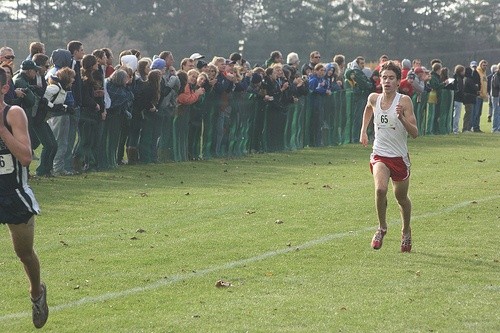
[34,161,129,179]
[453,125,483,134]
[491,129,500,133]
[487,115,492,122]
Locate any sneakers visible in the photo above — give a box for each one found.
[401,227,414,254]
[32,282,50,329]
[371,226,388,250]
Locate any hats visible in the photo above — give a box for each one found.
[198,61,207,68]
[20,60,41,70]
[190,53,205,61]
[151,58,166,70]
[225,59,237,65]
[471,60,477,67]
[414,67,423,73]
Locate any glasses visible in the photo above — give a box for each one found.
[313,57,321,59]
[0,55,15,60]
[42,63,51,69]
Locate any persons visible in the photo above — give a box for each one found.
[356,62,419,254]
[0,67,49,328]
[0,40,500,168]
